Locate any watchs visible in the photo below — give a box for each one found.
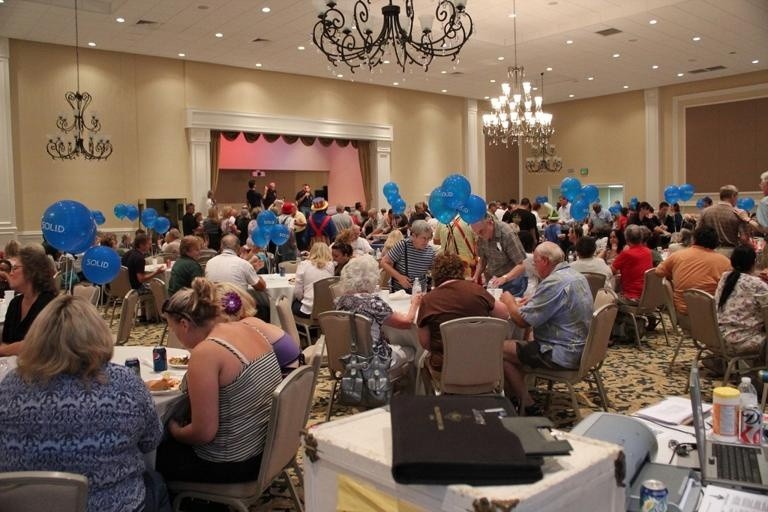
[502,275,509,282]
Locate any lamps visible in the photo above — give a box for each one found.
[45,3,113,161]
[526,71,563,174]
[483,1,554,148]
[311,0,474,73]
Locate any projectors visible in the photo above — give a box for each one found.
[252,169,265,176]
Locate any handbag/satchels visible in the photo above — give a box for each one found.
[451,218,482,286]
[336,307,393,410]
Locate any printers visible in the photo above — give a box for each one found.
[568,411,702,512]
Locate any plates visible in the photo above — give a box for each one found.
[146,378,182,396]
[168,358,188,369]
[288,279,296,285]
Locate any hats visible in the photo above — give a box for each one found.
[311,197,330,210]
[281,201,294,213]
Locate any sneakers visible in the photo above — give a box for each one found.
[514,404,545,417]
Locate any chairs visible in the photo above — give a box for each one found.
[682,289,761,396]
[592,287,618,310]
[435,315,510,398]
[276,294,330,367]
[108,288,139,345]
[277,260,303,272]
[1,470,90,512]
[315,310,416,420]
[292,275,341,347]
[582,271,608,287]
[169,358,316,512]
[105,263,157,329]
[149,278,173,344]
[521,301,619,419]
[72,282,101,309]
[661,275,692,375]
[617,267,671,352]
[306,335,326,366]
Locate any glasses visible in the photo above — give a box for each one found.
[415,232,433,244]
[9,264,25,273]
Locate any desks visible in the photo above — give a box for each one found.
[575,397,768,512]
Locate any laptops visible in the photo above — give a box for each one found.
[689,357,768,494]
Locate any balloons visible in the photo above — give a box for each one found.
[392,198,407,215]
[270,223,289,245]
[154,216,170,234]
[428,186,458,224]
[114,203,139,222]
[560,176,581,203]
[251,226,266,248]
[679,184,694,201]
[664,183,680,205]
[383,182,399,198]
[736,198,743,209]
[387,191,400,207]
[141,208,158,230]
[570,200,589,220]
[459,194,487,224]
[81,246,121,284]
[741,197,755,211]
[41,200,105,254]
[442,174,471,209]
[581,185,599,205]
[256,209,276,234]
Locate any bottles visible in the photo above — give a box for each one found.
[412,277,421,303]
[739,406,762,445]
[376,248,380,259]
[166,259,171,268]
[568,251,574,262]
[712,387,739,436]
[639,479,668,512]
[153,347,167,372]
[280,266,285,276]
[125,358,140,375]
[374,285,381,297]
[737,377,757,407]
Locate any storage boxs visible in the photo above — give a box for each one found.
[300,400,627,512]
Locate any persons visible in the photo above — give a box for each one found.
[3,239,21,258]
[666,228,692,257]
[628,201,650,230]
[569,237,613,288]
[470,212,529,296]
[351,224,373,254]
[182,190,254,234]
[508,197,537,252]
[1,295,162,512]
[194,232,217,273]
[567,224,583,244]
[558,195,571,220]
[278,202,297,260]
[707,244,768,356]
[331,243,353,276]
[486,198,517,223]
[517,230,540,341]
[307,197,336,250]
[291,203,307,248]
[295,184,314,218]
[217,281,300,371]
[640,225,663,267]
[127,235,166,295]
[499,242,594,416]
[699,185,754,258]
[161,277,283,484]
[544,211,565,242]
[96,228,181,265]
[612,207,629,232]
[530,203,542,244]
[607,229,629,258]
[669,203,682,232]
[433,213,478,278]
[335,254,423,380]
[292,242,335,319]
[332,201,353,234]
[654,225,734,331]
[264,182,277,210]
[611,224,654,306]
[651,202,674,249]
[702,197,712,207]
[409,201,429,235]
[381,220,436,295]
[0,246,60,354]
[0,258,12,297]
[362,208,408,241]
[245,179,268,210]
[733,171,768,243]
[416,254,510,397]
[205,234,270,324]
[168,235,204,296]
[588,203,613,237]
[353,202,364,227]
